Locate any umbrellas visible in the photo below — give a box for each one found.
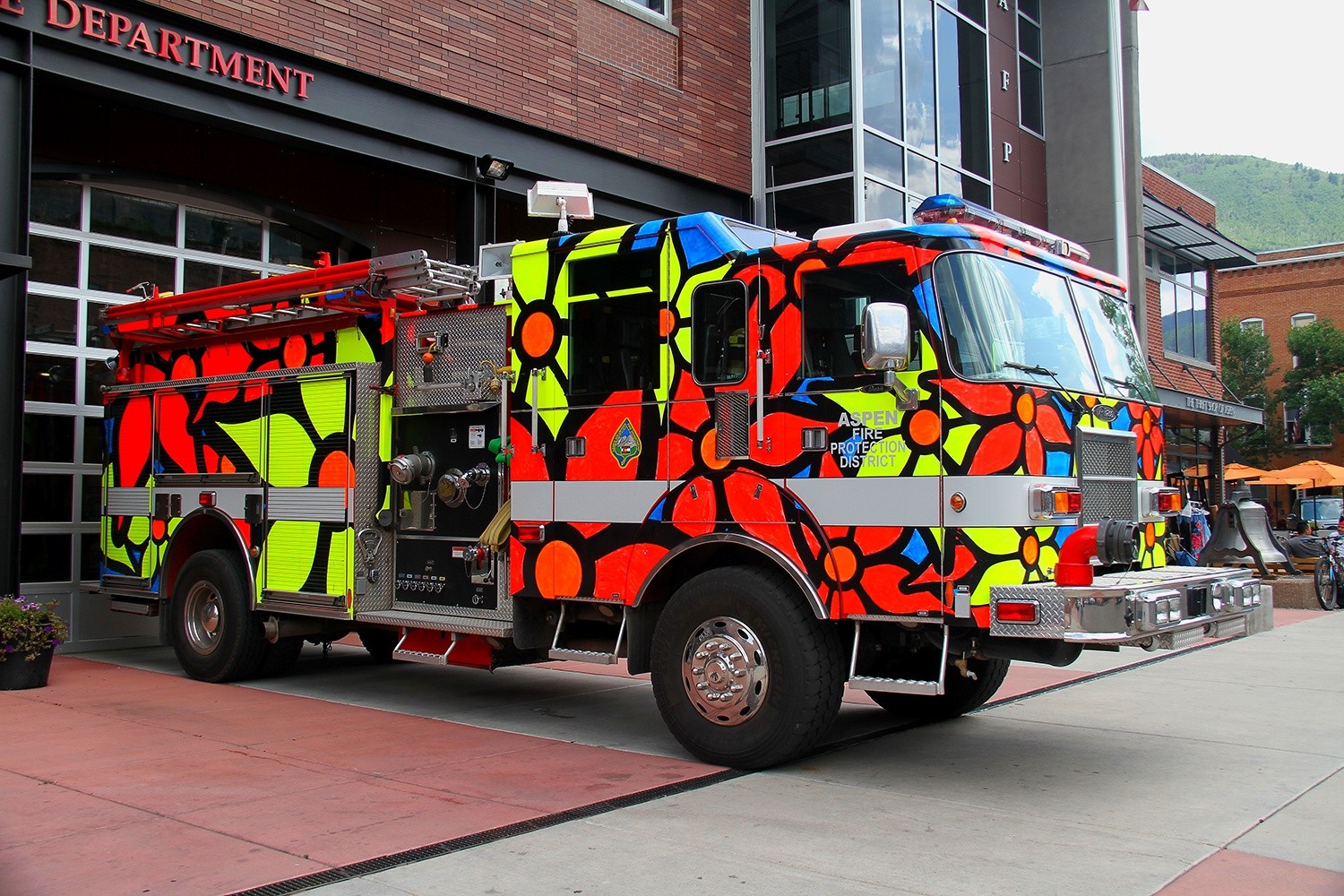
[1175,458,1344,530]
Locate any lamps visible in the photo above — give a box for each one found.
[479,154,515,181]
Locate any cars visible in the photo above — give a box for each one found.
[1285,497,1344,530]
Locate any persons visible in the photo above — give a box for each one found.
[1261,498,1344,574]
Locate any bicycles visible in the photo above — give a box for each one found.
[1305,534,1344,611]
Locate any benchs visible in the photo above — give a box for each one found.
[1212,557,1327,576]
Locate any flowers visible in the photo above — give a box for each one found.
[0,592,73,662]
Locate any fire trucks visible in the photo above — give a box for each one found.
[100,194,1273,774]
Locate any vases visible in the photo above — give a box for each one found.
[0,615,56,691]
